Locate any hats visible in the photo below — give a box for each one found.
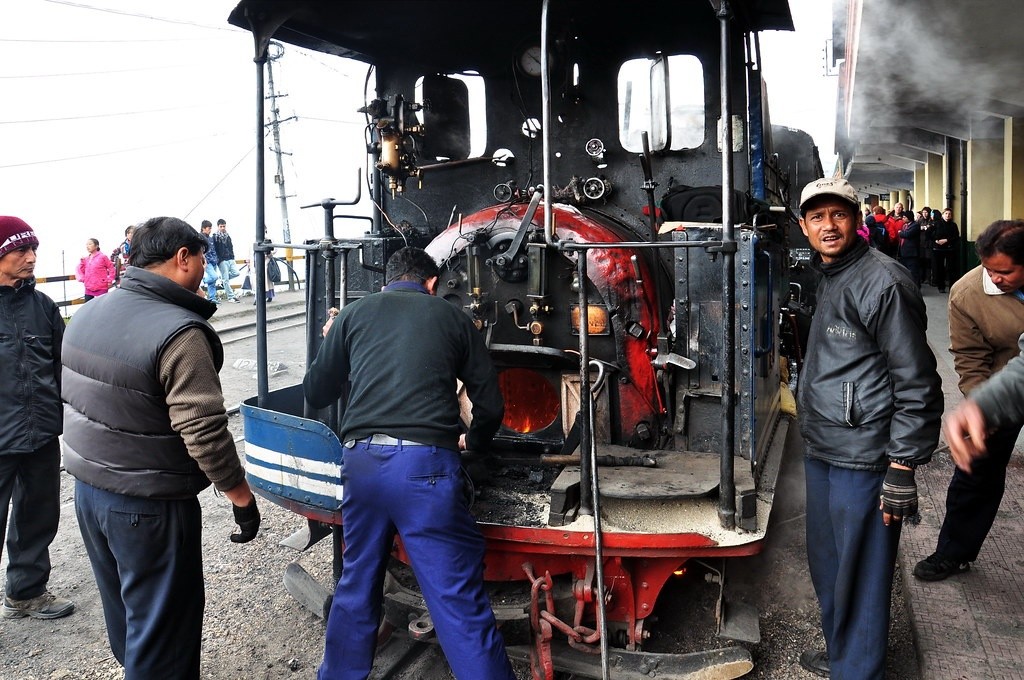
[903,211,914,221]
[800,176,862,214]
[865,216,876,226]
[0,216,40,259]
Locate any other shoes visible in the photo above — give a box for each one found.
[939,290,946,293]
[227,297,239,304]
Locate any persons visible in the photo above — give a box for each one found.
[60,217,260,680]
[916,220,1024,580]
[0,216,74,619]
[75,219,280,305]
[796,178,943,680]
[303,247,516,680]
[865,203,960,293]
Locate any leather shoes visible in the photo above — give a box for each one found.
[798,649,832,676]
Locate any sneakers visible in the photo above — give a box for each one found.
[914,548,968,581]
[3,590,76,620]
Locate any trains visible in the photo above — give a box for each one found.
[224,1,830,679]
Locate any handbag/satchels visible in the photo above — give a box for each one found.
[241,276,253,289]
[268,257,282,281]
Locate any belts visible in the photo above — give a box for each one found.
[343,433,427,449]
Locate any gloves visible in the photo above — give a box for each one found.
[230,493,261,543]
